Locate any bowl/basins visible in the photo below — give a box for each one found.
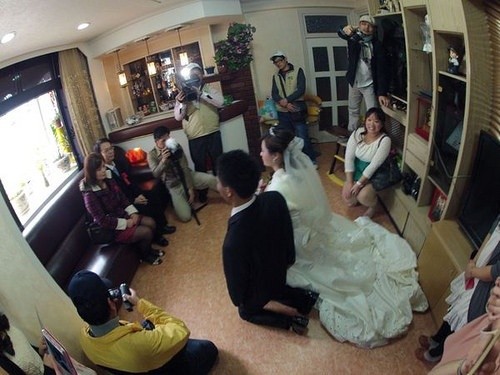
[126,99,176,126]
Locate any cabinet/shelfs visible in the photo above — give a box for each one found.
[366,0,500,329]
[121,40,206,118]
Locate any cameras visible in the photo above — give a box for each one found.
[350,29,364,44]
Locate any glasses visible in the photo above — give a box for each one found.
[273,58,284,64]
[101,147,114,153]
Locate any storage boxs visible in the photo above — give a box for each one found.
[105,106,124,131]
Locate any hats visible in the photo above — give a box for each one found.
[185,62,203,79]
[269,49,287,61]
[359,15,376,26]
[67,269,115,306]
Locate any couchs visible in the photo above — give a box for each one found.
[22,163,172,319]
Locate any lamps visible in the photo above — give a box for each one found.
[139,35,160,77]
[125,148,147,164]
[112,48,129,88]
[175,27,188,66]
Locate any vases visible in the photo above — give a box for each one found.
[204,66,216,75]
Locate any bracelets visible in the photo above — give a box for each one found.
[355,180,364,188]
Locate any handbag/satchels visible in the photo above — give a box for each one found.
[87,221,116,245]
[370,134,402,192]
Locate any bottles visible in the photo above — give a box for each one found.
[129,54,176,98]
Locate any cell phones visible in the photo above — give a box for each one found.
[39,329,78,375]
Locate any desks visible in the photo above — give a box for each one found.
[265,115,320,127]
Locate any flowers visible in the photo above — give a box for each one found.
[212,21,257,74]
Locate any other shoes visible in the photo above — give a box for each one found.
[418,335,437,349]
[292,314,309,336]
[152,234,169,247]
[414,346,441,364]
[297,290,320,315]
[157,225,176,234]
[199,190,208,202]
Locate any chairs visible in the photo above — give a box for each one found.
[305,95,322,142]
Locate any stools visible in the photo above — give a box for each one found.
[330,135,348,178]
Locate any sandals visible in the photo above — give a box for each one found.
[152,249,166,257]
[138,254,162,266]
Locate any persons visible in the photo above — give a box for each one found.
[214,149,322,335]
[341,106,393,221]
[80,153,166,267]
[254,124,339,285]
[146,125,221,222]
[269,52,321,171]
[428,277,500,375]
[68,271,218,375]
[94,137,177,247]
[335,13,391,138]
[414,209,500,365]
[174,62,227,211]
[0,314,58,375]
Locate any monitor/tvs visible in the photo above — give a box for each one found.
[457,131,500,251]
